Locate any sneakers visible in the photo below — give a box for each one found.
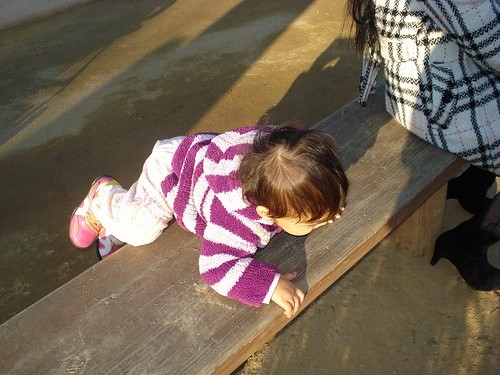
[68,174,125,261]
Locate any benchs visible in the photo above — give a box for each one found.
[1,81,470,375]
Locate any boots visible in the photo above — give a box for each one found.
[429,165,500,292]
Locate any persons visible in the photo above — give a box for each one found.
[347,0,500,292]
[68,124,350,318]
